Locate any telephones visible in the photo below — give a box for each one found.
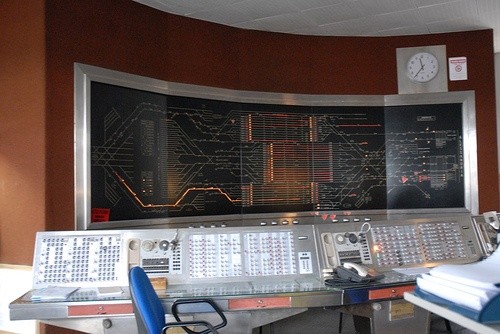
[336,262,385,284]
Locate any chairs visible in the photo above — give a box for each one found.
[126,266,228,334]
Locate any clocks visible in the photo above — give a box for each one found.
[407,51,441,83]
